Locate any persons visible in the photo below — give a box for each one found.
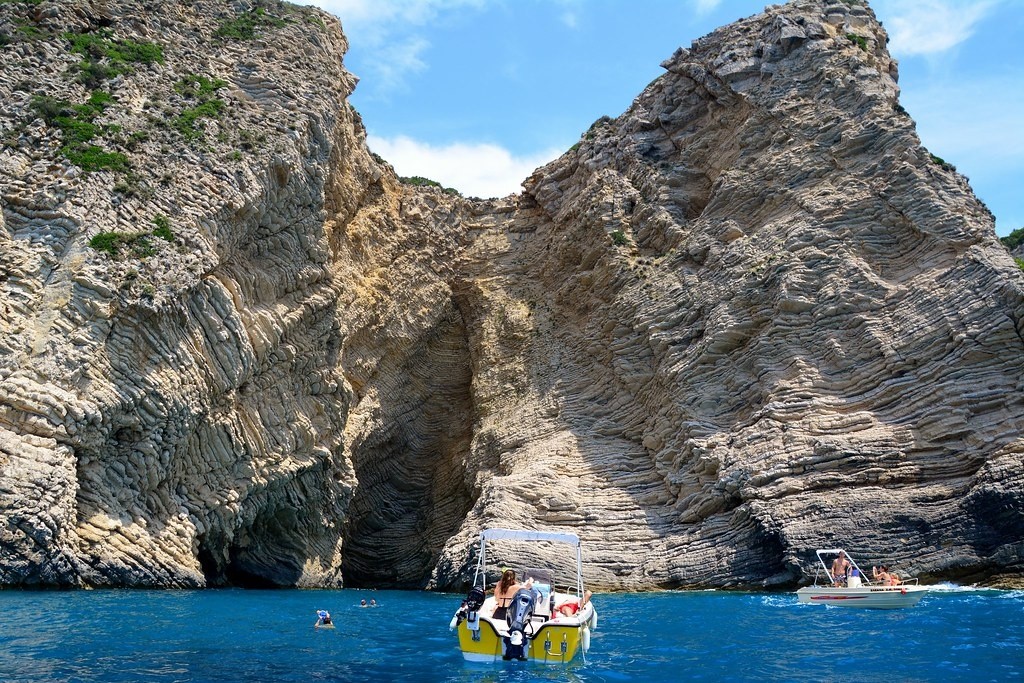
[491,569,536,620]
[370,597,377,607]
[873,565,891,591]
[314,616,333,628]
[831,550,852,588]
[360,598,367,607]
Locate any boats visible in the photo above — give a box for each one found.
[796,549,930,609]
[314,619,336,629]
[449,527,598,665]
